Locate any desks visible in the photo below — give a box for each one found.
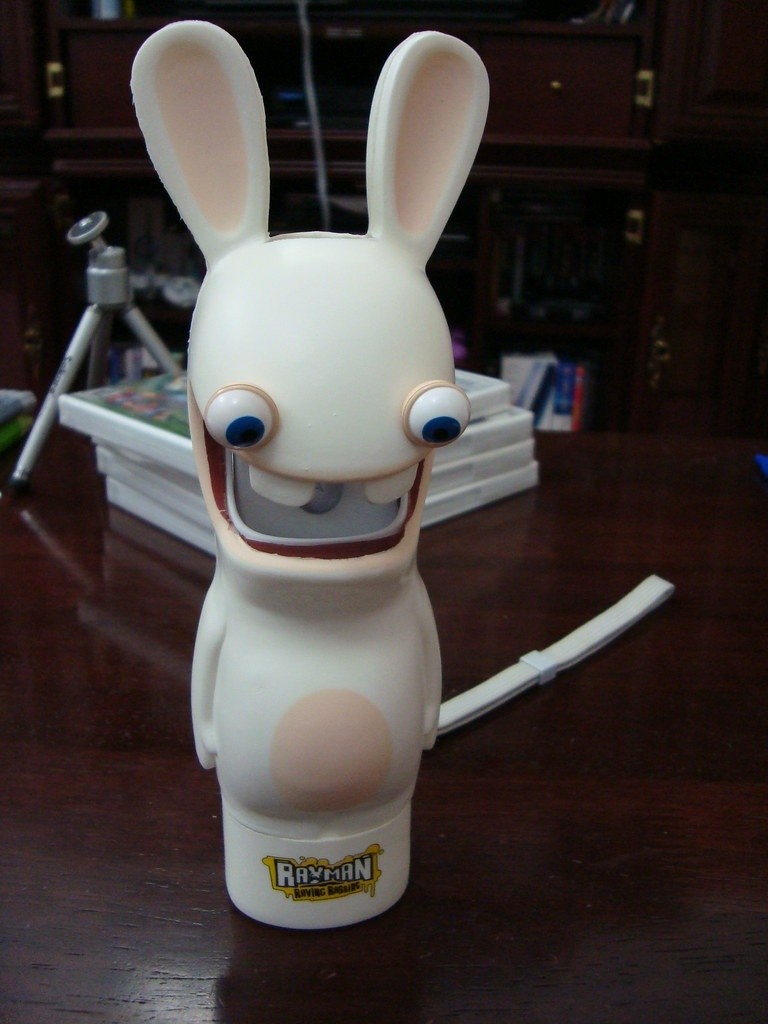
[0,397,766,1024]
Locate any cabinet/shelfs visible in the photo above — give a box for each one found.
[0,1,768,436]
